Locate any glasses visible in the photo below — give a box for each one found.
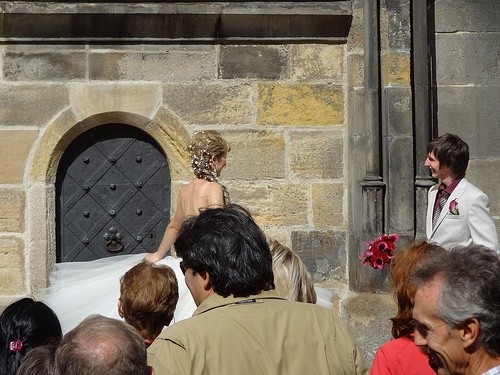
[180,261,194,276]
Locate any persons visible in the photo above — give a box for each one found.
[425,132,499,256]
[146,205,368,375]
[18,344,61,375]
[41,129,330,333]
[411,244,500,375]
[369,242,445,375]
[0,297,62,375]
[56,316,156,375]
[118,263,178,347]
[266,236,317,305]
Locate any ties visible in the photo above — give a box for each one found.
[432,191,448,231]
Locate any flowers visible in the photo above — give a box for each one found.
[358,234,400,270]
